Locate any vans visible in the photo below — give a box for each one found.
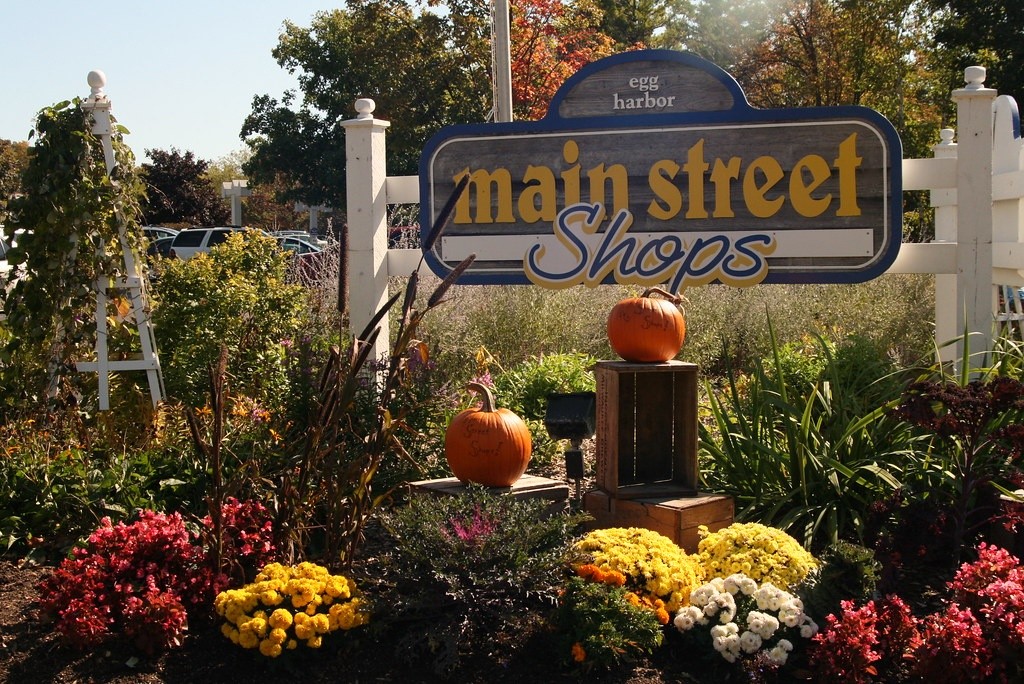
[169,225,273,261]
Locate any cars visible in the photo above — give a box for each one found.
[271,230,341,255]
[140,226,180,240]
[147,236,176,265]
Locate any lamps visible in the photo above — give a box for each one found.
[543,389,597,501]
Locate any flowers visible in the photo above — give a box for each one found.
[571,520,824,671]
[36,498,371,661]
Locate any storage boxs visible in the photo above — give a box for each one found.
[595,359,699,498]
[584,488,735,555]
[410,471,571,522]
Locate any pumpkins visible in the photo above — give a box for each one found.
[445,380,532,487]
[608,287,686,364]
